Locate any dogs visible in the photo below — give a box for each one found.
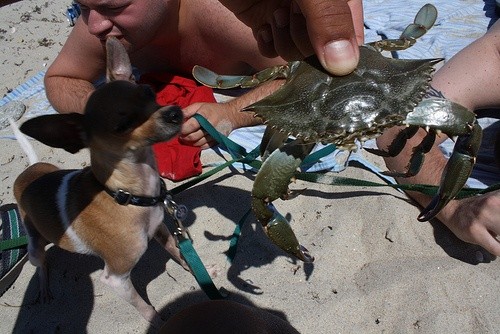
[14,35,184,330]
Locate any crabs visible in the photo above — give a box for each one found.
[192,3,484,261]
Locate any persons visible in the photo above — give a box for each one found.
[218,1,360,76]
[44,0,365,150]
[374,0,500,257]
